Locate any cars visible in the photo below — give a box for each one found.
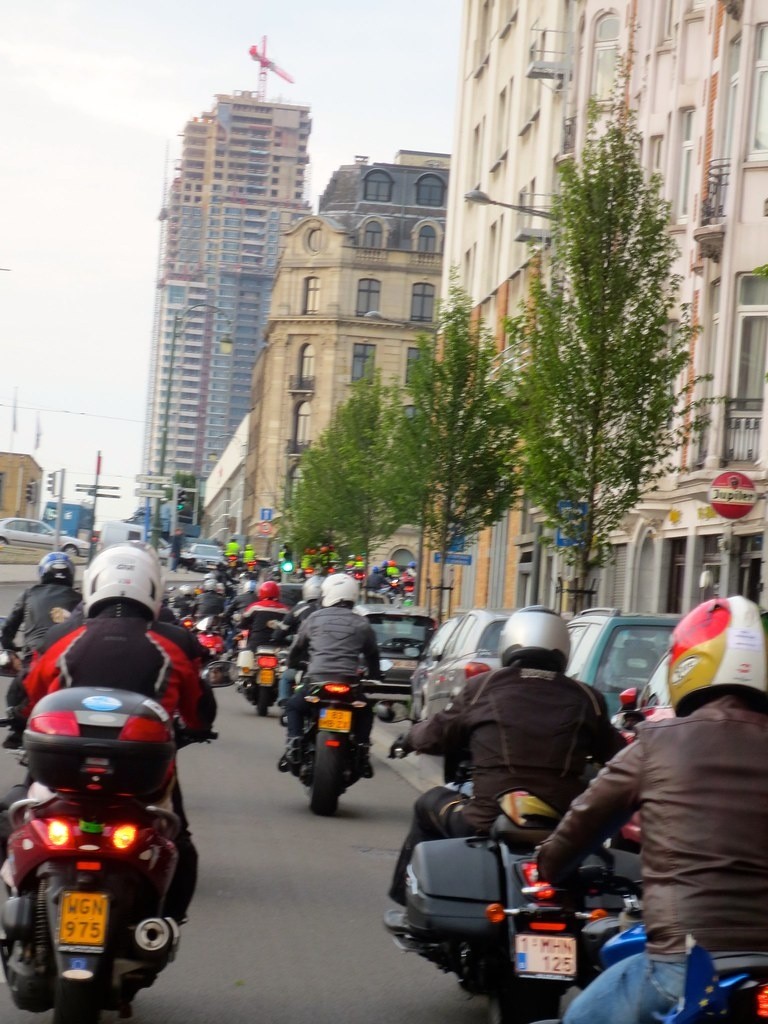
[351,603,438,702]
[0,517,91,557]
[188,543,224,571]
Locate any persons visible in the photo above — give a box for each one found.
[537,591,768,1024]
[383,605,628,934]
[222,537,417,605]
[0,541,218,926]
[0,552,379,820]
[169,528,186,573]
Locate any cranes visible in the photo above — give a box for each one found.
[250,39,294,102]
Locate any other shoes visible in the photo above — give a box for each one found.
[384,909,431,941]
[278,752,294,773]
[352,756,373,778]
[169,570,177,573]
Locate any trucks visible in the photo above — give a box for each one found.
[91,519,171,566]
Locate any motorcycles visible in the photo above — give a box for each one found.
[173,557,414,816]
[0,604,219,1024]
[383,732,768,1024]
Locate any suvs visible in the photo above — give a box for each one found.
[409,607,524,725]
[565,607,685,720]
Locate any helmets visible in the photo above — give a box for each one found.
[500,604,572,671]
[81,546,162,628]
[246,544,253,550]
[321,574,359,608]
[372,567,378,572]
[668,596,768,716]
[409,562,416,568]
[203,580,216,590]
[382,561,388,567]
[182,585,193,595]
[38,552,74,587]
[389,560,396,566]
[259,581,280,600]
[303,576,325,603]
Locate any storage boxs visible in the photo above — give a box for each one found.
[22,687,174,795]
[402,836,501,952]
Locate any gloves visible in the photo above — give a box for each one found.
[389,735,412,759]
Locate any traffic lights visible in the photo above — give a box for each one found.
[25,483,32,501]
[280,560,293,573]
[47,472,54,492]
[176,489,187,511]
